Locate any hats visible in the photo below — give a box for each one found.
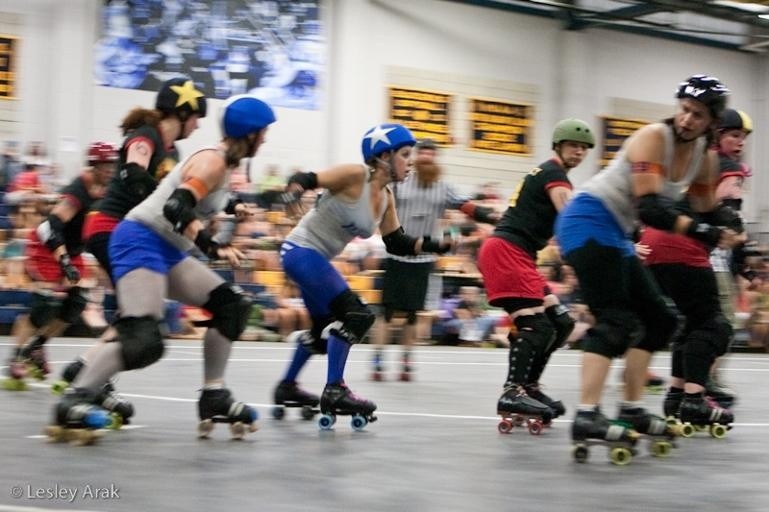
[417,138,436,149]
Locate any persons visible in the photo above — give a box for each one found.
[61,78,206,420]
[55,94,275,429]
[554,75,749,446]
[9,142,120,377]
[373,139,505,374]
[0,140,769,354]
[708,244,737,401]
[479,119,653,422]
[638,110,764,426]
[275,124,459,414]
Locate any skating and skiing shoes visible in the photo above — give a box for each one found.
[497,385,554,434]
[319,379,377,430]
[1,335,133,446]
[274,379,320,420]
[571,366,735,464]
[525,381,565,427]
[197,389,258,440]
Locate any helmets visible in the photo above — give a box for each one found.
[156,78,206,118]
[676,74,728,118]
[224,98,276,138]
[551,118,595,150]
[362,124,416,165]
[716,107,753,133]
